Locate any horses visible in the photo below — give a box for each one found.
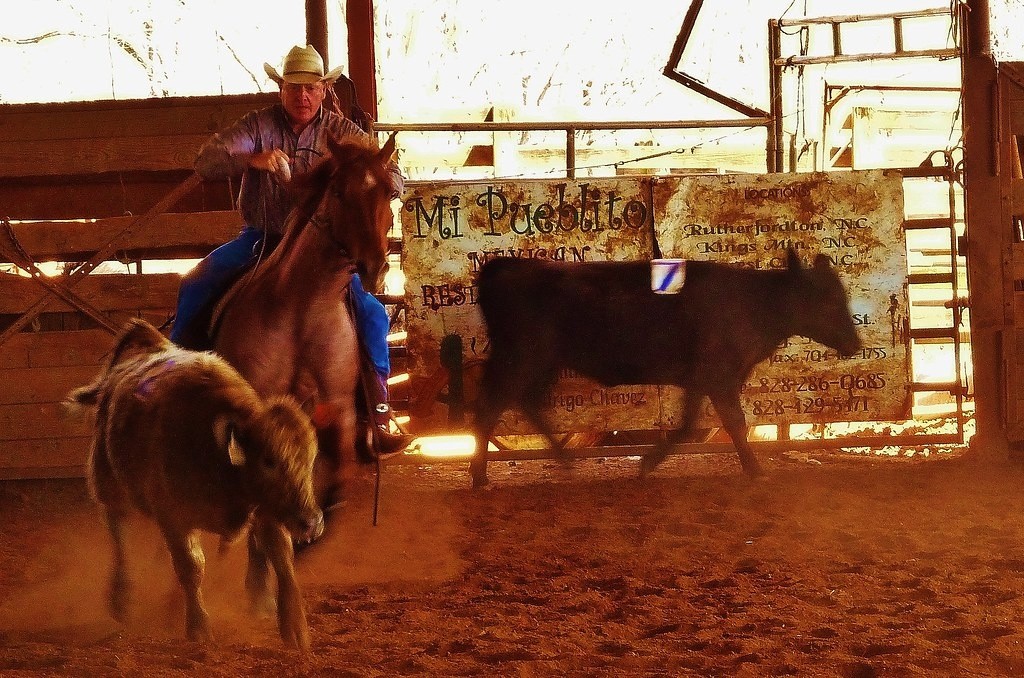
[204,130,401,627]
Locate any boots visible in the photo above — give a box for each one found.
[366,371,414,451]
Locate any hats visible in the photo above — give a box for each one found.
[263,45,344,84]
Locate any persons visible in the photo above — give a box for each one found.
[169,45,418,454]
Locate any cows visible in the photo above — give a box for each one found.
[69,317,325,655]
[469,240,861,489]
